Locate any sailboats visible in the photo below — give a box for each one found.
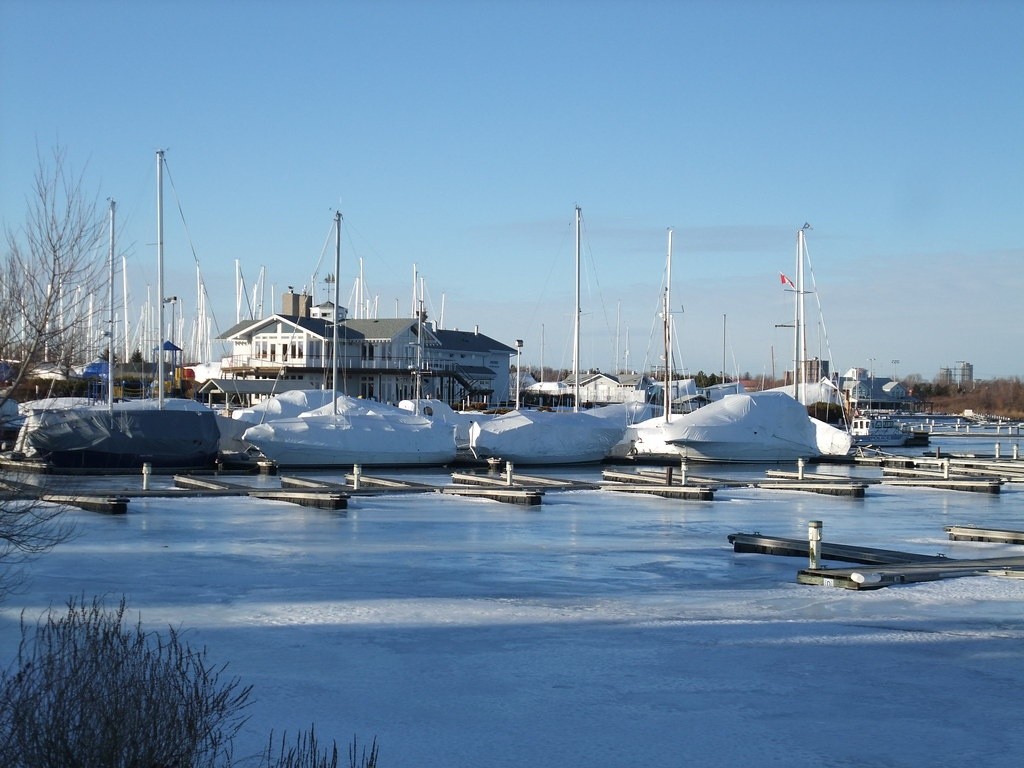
[466,205,630,464]
[14,145,839,454]
[15,149,221,470]
[239,209,459,467]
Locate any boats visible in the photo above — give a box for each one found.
[843,416,911,448]
[666,388,820,461]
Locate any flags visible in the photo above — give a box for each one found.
[803,222,814,231]
[781,272,794,288]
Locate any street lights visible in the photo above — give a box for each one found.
[867,358,876,415]
[515,340,523,410]
[161,296,177,393]
[891,360,900,412]
